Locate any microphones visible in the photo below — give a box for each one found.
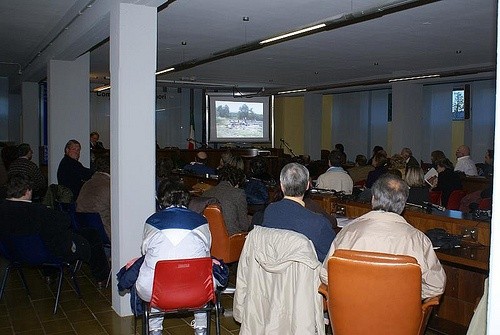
[280,139,284,143]
[187,138,192,141]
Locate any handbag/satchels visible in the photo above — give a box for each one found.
[238,180,269,205]
[424,228,463,248]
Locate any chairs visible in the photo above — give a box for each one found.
[132,257,225,335]
[0,184,111,315]
[426,190,442,206]
[318,248,442,335]
[188,197,249,294]
[444,189,466,210]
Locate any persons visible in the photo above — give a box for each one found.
[335,143,344,152]
[432,158,459,191]
[454,145,477,176]
[221,150,268,204]
[262,163,336,263]
[347,146,419,188]
[57,140,91,198]
[480,150,493,176]
[430,151,446,169]
[246,156,278,182]
[3,174,110,286]
[90,132,105,154]
[185,151,217,175]
[76,149,111,240]
[135,180,215,335]
[320,173,447,300]
[9,143,46,187]
[404,166,429,203]
[203,166,249,235]
[315,151,353,195]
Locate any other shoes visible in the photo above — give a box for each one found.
[194,327,207,335]
[149,329,163,335]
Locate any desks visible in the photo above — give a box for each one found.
[87,148,493,328]
